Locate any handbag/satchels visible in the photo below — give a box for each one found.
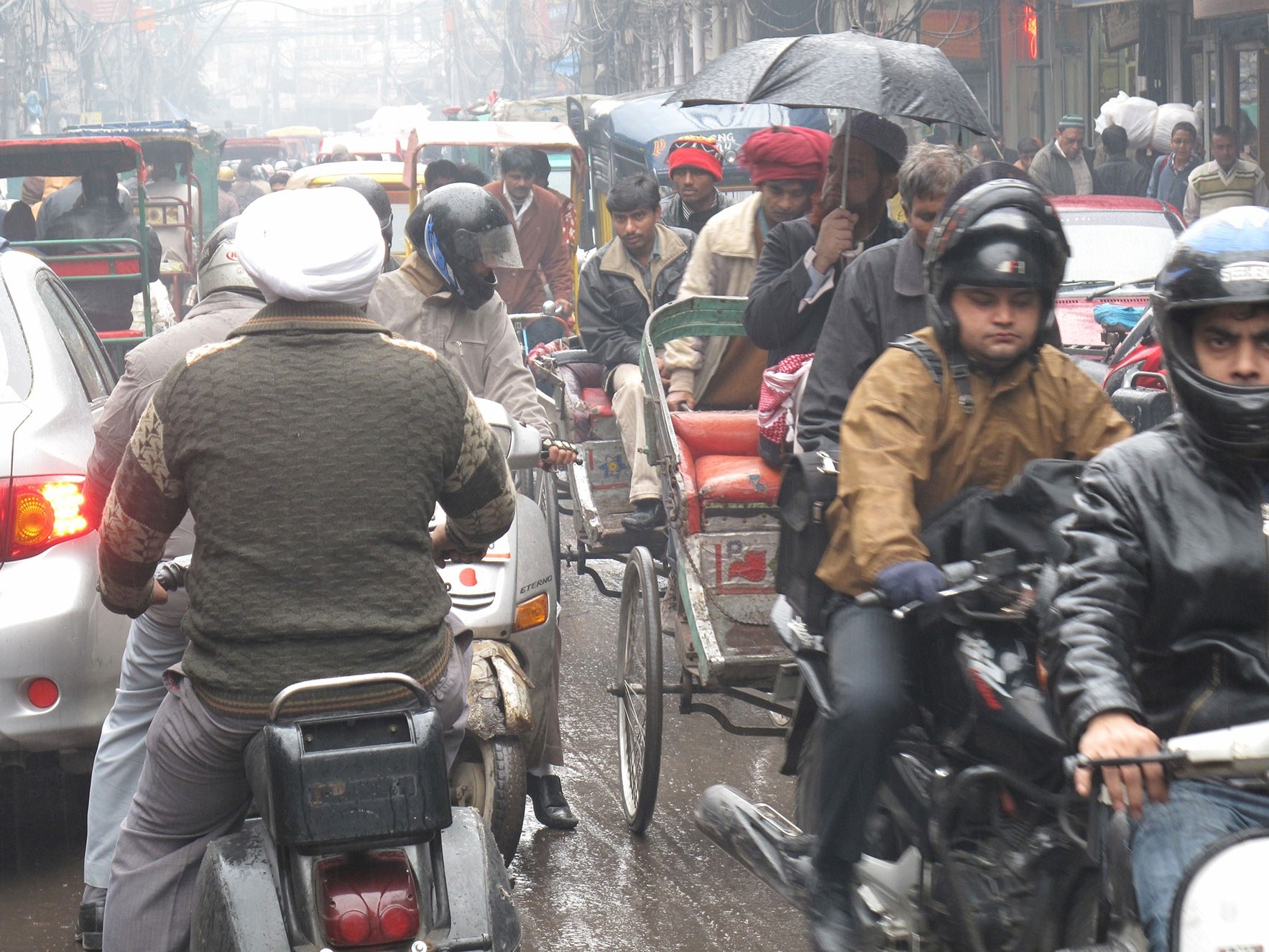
[771,448,848,637]
[752,349,810,468]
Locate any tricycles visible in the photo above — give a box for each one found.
[1,120,225,383]
[588,89,797,249]
[284,158,439,264]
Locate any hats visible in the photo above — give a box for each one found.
[666,136,727,185]
[1058,114,1086,133]
[835,111,911,166]
[743,124,828,187]
[274,161,288,170]
[234,179,391,307]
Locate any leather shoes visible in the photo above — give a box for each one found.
[622,508,656,534]
[72,897,104,951]
[524,770,580,828]
[804,865,861,952]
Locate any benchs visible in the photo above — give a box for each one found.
[562,360,642,517]
[666,406,788,537]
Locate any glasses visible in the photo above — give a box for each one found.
[1171,137,1193,146]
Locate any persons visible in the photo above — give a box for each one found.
[734,115,912,468]
[0,87,1268,284]
[576,176,701,537]
[73,213,250,952]
[1037,196,1269,949]
[364,181,587,835]
[793,157,1136,951]
[778,142,987,458]
[99,181,520,952]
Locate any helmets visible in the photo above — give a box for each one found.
[1149,202,1269,463]
[329,175,397,270]
[194,215,267,300]
[288,159,303,172]
[401,185,520,315]
[217,165,237,182]
[919,161,1069,350]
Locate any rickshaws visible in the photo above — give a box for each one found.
[526,325,669,602]
[404,119,588,421]
[605,296,798,838]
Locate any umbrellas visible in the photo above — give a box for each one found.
[666,25,1005,255]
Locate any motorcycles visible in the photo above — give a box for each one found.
[426,395,590,872]
[147,524,534,950]
[692,550,1056,951]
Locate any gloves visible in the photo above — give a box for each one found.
[877,558,953,629]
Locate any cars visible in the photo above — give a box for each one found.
[1047,190,1189,346]
[1062,715,1269,952]
[0,252,152,795]
